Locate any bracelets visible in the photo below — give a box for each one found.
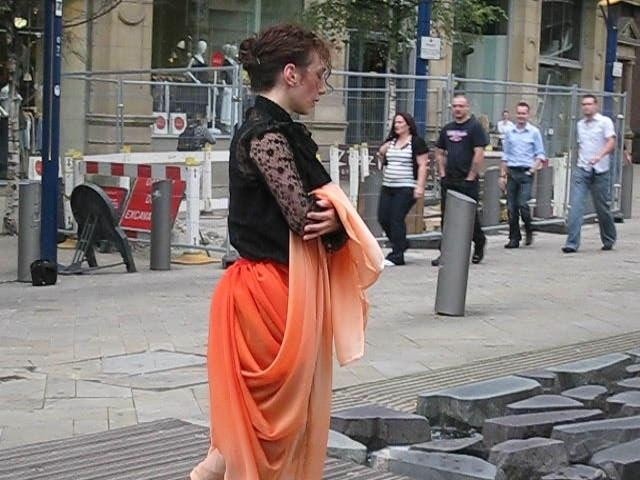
[377,151,385,160]
[499,175,507,181]
[526,169,534,177]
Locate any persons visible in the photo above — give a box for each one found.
[178,111,216,218]
[184,20,353,480]
[495,110,516,152]
[562,93,617,255]
[433,96,495,265]
[612,133,632,224]
[168,33,243,136]
[375,109,429,267]
[478,112,497,150]
[498,100,547,248]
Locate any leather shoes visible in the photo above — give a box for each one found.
[383,258,395,266]
[472,240,485,263]
[601,246,611,251]
[561,247,575,252]
[505,241,519,248]
[431,255,441,265]
[526,232,531,245]
